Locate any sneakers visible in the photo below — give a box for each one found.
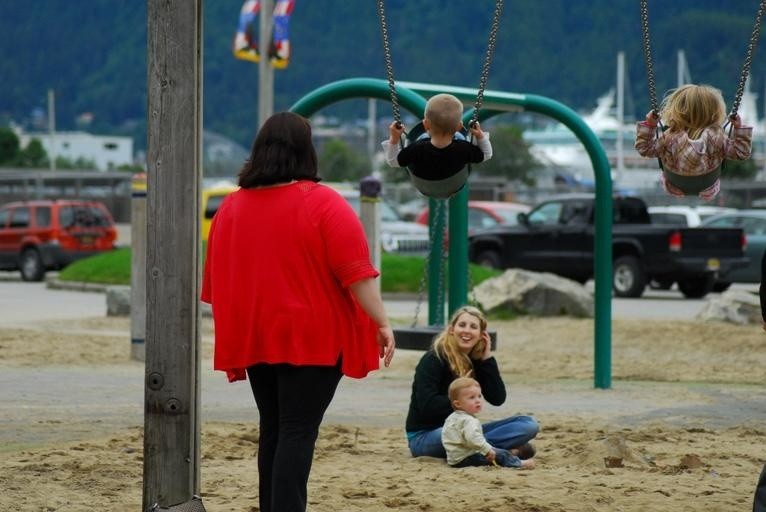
[512,439,537,461]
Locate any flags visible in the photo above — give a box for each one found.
[230,0,296,70]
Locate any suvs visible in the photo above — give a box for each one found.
[0,195,120,283]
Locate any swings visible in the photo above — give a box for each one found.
[378,0,504,196]
[640,0,765,199]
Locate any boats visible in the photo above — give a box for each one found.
[516,42,766,183]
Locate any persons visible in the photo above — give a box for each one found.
[438,375,537,470]
[201,109,398,510]
[405,304,542,460]
[378,92,494,200]
[633,83,754,202]
